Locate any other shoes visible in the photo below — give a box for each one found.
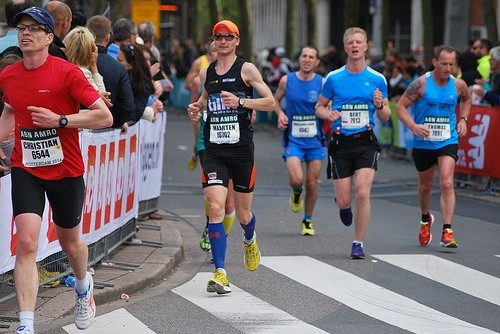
[148,214,161,220]
[63,263,95,276]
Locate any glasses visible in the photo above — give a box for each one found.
[123,44,135,57]
[472,46,482,50]
[214,32,238,41]
[15,23,52,34]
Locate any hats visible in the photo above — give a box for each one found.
[11,6,55,32]
[213,20,240,36]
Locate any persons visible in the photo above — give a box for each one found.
[186,20,276,295]
[0,0,500,287]
[0,6,113,334]
[274,45,331,236]
[395,46,472,247]
[313,27,391,259]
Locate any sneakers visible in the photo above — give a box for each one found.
[8,266,62,287]
[440,228,458,248]
[199,224,215,252]
[290,181,303,213]
[244,231,261,271]
[301,219,315,236]
[74,271,96,330]
[340,206,353,227]
[206,270,232,294]
[419,214,434,247]
[351,243,366,259]
[15,324,35,334]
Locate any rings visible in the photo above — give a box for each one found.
[190,112,193,115]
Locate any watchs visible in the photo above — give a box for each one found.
[374,102,384,110]
[237,97,245,108]
[460,117,468,122]
[59,114,68,129]
